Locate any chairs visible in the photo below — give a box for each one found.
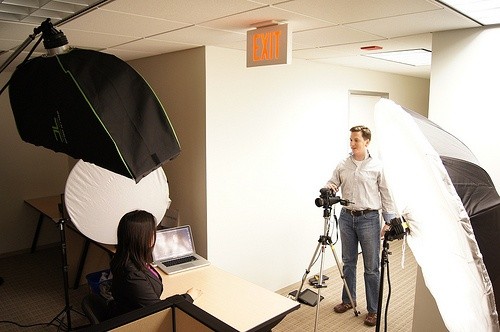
[81,294,108,326]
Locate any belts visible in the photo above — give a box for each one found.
[342,207,378,217]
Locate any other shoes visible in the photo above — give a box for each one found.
[334,302,356,312]
[364,313,377,326]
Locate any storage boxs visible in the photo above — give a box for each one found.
[86,269,114,294]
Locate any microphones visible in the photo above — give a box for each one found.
[315,196,339,207]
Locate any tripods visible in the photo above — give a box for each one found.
[45,221,93,330]
[294,207,357,332]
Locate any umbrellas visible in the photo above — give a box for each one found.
[374,99,500,332]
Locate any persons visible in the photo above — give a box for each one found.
[110,210,203,320]
[326,126,397,326]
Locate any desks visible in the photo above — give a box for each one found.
[25,195,300,332]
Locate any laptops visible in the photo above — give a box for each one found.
[151,225,210,275]
